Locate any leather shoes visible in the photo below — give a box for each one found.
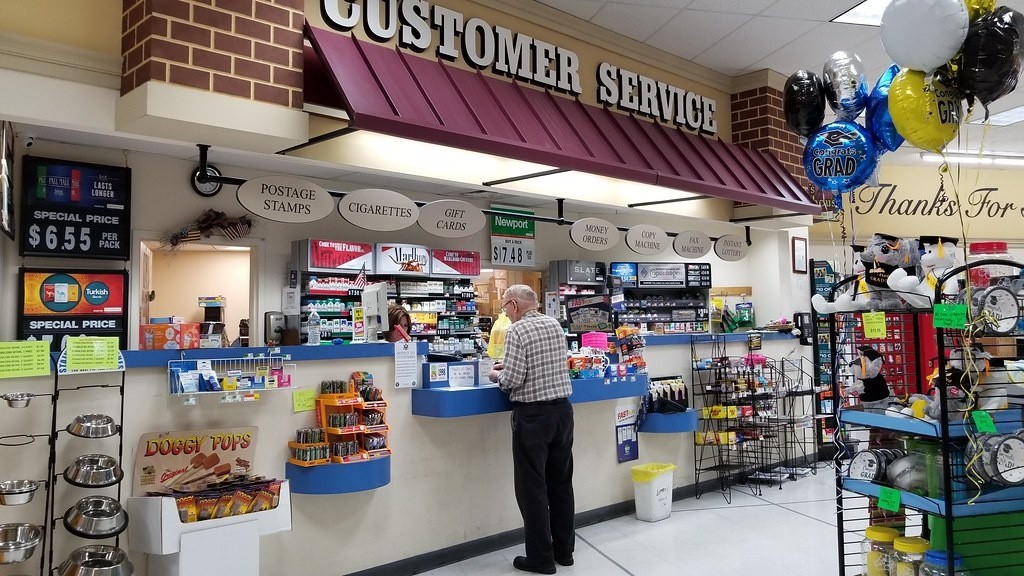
[513,556,556,575]
[554,553,574,566]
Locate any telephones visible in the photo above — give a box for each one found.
[793,312,812,340]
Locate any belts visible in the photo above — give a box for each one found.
[523,398,568,406]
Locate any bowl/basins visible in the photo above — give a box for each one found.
[963,428,1024,487]
[0,480,41,506]
[955,286,1020,337]
[0,523,45,565]
[847,447,928,498]
[2,392,35,409]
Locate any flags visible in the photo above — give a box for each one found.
[179,209,218,243]
[220,217,252,240]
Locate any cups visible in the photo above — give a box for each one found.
[593,355,610,378]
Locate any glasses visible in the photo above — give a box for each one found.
[500,299,517,312]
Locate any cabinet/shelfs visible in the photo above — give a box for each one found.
[283,266,487,344]
[610,285,712,335]
[823,258,1024,575]
[689,335,826,505]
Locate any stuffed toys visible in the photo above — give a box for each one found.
[811,232,959,314]
[885,343,992,423]
[843,345,895,415]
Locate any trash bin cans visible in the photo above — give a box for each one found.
[630,462,675,522]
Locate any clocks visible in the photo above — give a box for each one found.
[191,164,224,197]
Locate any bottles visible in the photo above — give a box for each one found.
[307,309,321,346]
[860,525,973,576]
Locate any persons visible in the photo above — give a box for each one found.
[488,284,575,575]
[382,304,412,343]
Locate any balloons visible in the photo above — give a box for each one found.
[783,0,1023,209]
